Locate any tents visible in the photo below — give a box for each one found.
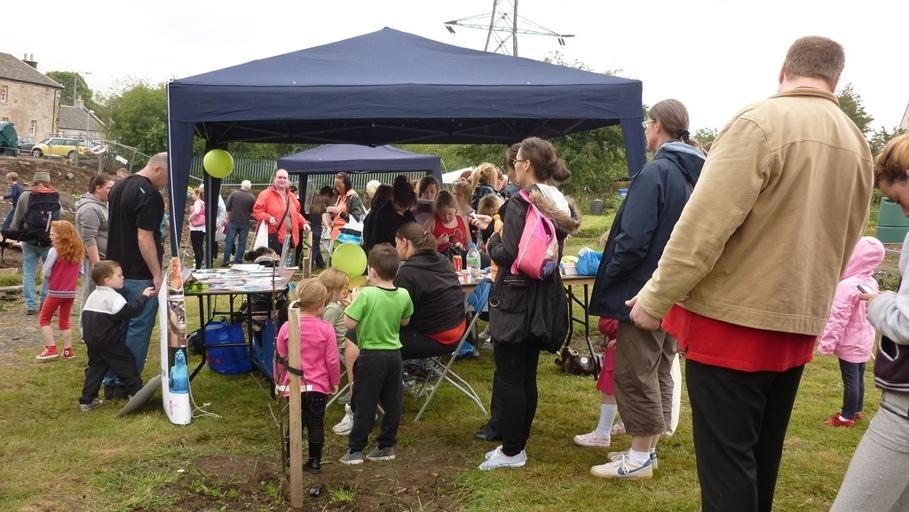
[167,27,647,271]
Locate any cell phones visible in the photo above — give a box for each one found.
[857,283,875,296]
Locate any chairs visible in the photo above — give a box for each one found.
[413,276,493,423]
[325,370,385,416]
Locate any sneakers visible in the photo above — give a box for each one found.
[338,390,352,403]
[61,345,75,359]
[479,443,527,470]
[365,444,396,461]
[480,340,495,351]
[103,383,113,399]
[820,407,862,429]
[78,399,105,411]
[114,384,130,399]
[304,457,320,475]
[606,451,659,471]
[590,453,653,481]
[338,449,365,467]
[331,402,354,436]
[573,428,612,450]
[478,324,491,338]
[34,345,59,361]
[610,421,626,436]
[475,424,502,440]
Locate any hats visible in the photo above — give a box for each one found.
[30,170,51,183]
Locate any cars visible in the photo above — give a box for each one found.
[85,138,109,157]
[16,138,46,152]
[32,138,85,159]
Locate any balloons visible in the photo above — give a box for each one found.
[560,255,580,263]
[203,149,234,178]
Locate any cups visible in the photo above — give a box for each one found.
[454,255,462,272]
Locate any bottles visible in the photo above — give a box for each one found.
[466,243,481,278]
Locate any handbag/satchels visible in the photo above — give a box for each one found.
[266,232,281,253]
[563,353,604,378]
[575,247,602,279]
[190,205,209,227]
[199,318,253,375]
[251,219,270,254]
[331,219,363,255]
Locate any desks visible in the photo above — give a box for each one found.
[455,272,480,293]
[184,269,296,402]
[558,271,599,382]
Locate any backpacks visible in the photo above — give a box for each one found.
[22,191,61,248]
[510,191,560,281]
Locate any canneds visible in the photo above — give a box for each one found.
[452,255,463,272]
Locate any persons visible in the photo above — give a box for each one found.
[818,235,886,426]
[272,279,341,473]
[572,317,626,448]
[828,134,909,512]
[339,243,414,464]
[333,221,466,435]
[624,36,875,512]
[479,136,581,471]
[613,355,683,436]
[1,151,169,409]
[170,152,511,411]
[475,142,522,441]
[588,99,708,480]
